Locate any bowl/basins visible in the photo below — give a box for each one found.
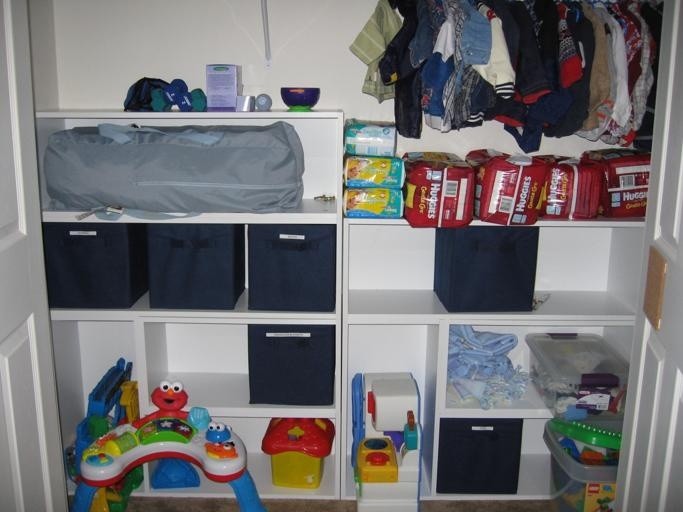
[280,87,320,113]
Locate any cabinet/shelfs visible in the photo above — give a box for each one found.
[0,0,682,512]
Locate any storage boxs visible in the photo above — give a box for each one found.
[247,324,336,405]
[437,334,630,512]
[146,224,245,312]
[247,223,336,313]
[41,222,149,311]
[434,226,540,313]
